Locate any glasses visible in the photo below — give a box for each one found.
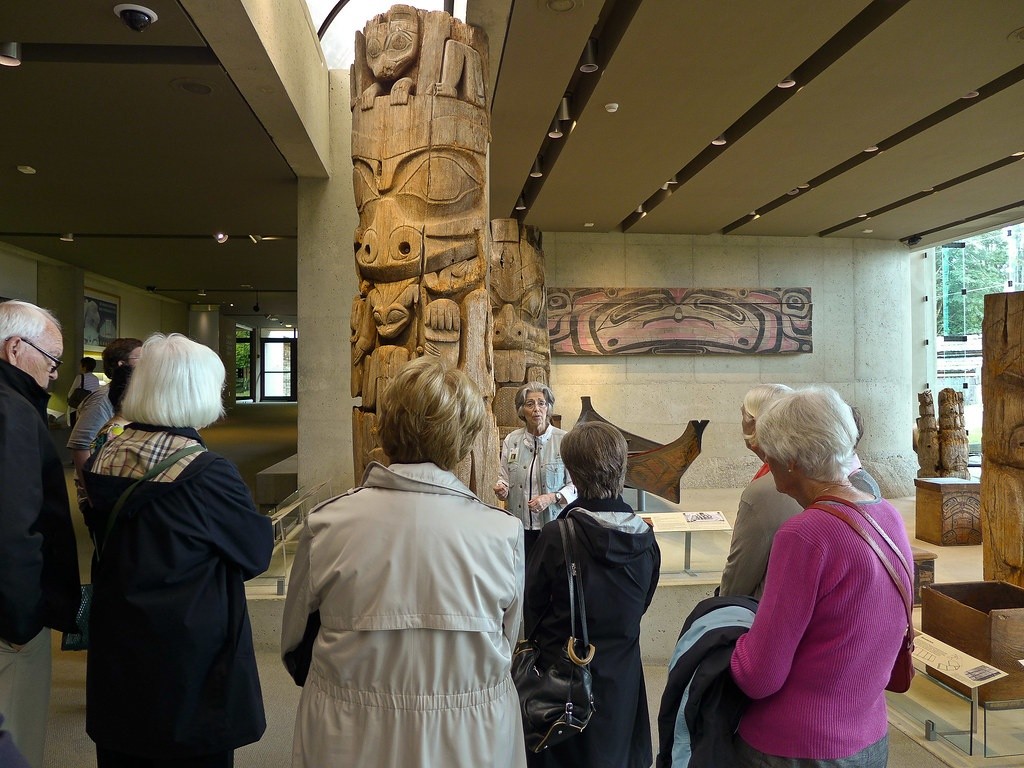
[6,335,64,374]
[524,399,547,409]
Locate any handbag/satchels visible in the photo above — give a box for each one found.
[884,630,915,694]
[510,518,596,755]
[60,584,89,651]
[68,374,92,409]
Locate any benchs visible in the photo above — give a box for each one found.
[256,451,298,515]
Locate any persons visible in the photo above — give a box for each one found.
[281,355,526,768]
[0,299,82,768]
[848,406,881,497]
[83,332,274,768]
[526,421,661,768]
[718,384,805,602]
[64,337,143,560]
[494,381,578,560]
[730,384,914,768]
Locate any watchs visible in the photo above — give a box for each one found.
[554,492,562,504]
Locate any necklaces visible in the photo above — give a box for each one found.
[814,481,852,499]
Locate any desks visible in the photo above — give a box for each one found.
[910,544,938,608]
[914,478,985,547]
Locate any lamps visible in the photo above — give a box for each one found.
[249,234,262,243]
[548,119,563,139]
[712,133,726,146]
[580,38,599,73]
[214,234,228,243]
[635,204,643,213]
[557,98,572,121]
[778,72,796,89]
[198,289,205,295]
[0,41,22,67]
[529,157,544,177]
[60,233,74,241]
[661,174,678,190]
[515,192,526,211]
